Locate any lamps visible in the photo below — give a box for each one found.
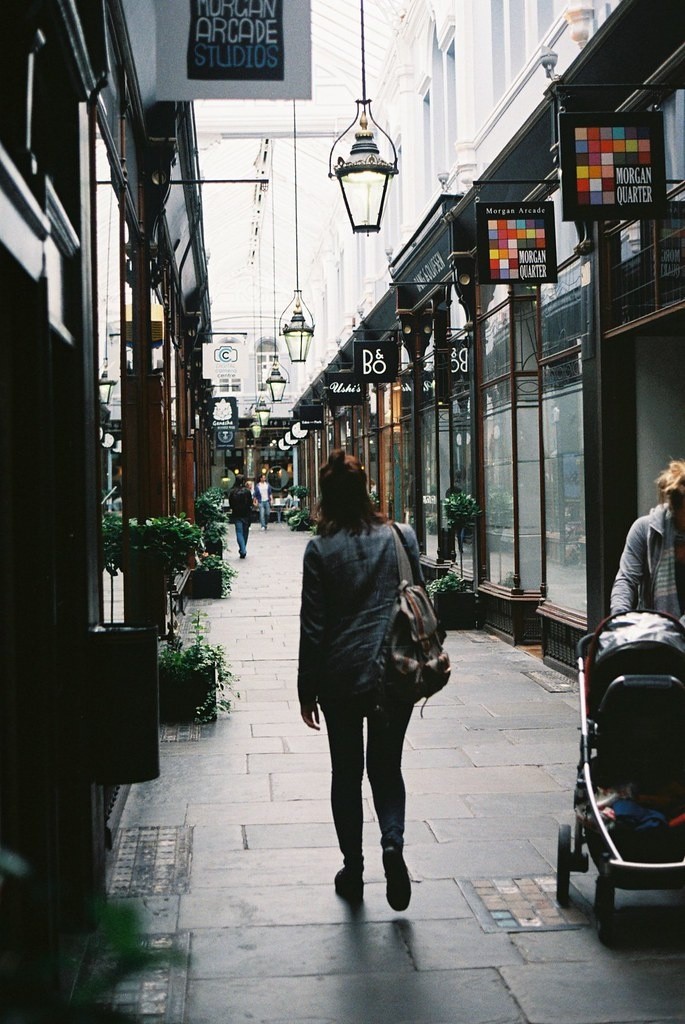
[384,247,394,263]
[249,417,262,438]
[255,310,271,427]
[279,99,315,361]
[336,339,342,348]
[97,188,118,404]
[265,209,290,401]
[437,172,451,191]
[540,44,563,81]
[329,0,398,233]
[357,308,365,320]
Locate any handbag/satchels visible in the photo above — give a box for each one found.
[385,521,447,644]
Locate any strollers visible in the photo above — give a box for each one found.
[555,609,685,934]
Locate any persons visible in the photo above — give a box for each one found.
[229,473,254,560]
[295,448,451,915]
[608,454,685,629]
[252,472,273,532]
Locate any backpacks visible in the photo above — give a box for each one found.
[384,522,452,699]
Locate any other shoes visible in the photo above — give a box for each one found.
[335,867,364,898]
[383,840,411,911]
[261,526,267,531]
[239,550,246,558]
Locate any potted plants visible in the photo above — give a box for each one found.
[425,572,478,630]
[158,607,241,725]
[201,521,229,560]
[193,553,238,599]
[289,508,311,531]
